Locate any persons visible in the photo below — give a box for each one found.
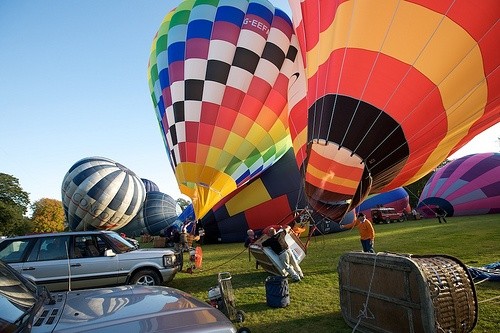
[172,228,189,252]
[402,207,417,222]
[244,229,257,247]
[252,226,304,281]
[435,205,448,223]
[339,213,375,252]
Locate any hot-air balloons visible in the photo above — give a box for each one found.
[147,0,308,222]
[248,0,500,276]
[115,179,183,238]
[60,156,146,233]
[165,146,500,244]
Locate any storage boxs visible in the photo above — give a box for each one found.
[142,234,165,247]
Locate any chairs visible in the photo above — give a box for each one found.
[39,239,100,258]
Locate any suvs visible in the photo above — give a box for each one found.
[0,231,181,292]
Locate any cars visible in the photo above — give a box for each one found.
[0,261,238,333]
